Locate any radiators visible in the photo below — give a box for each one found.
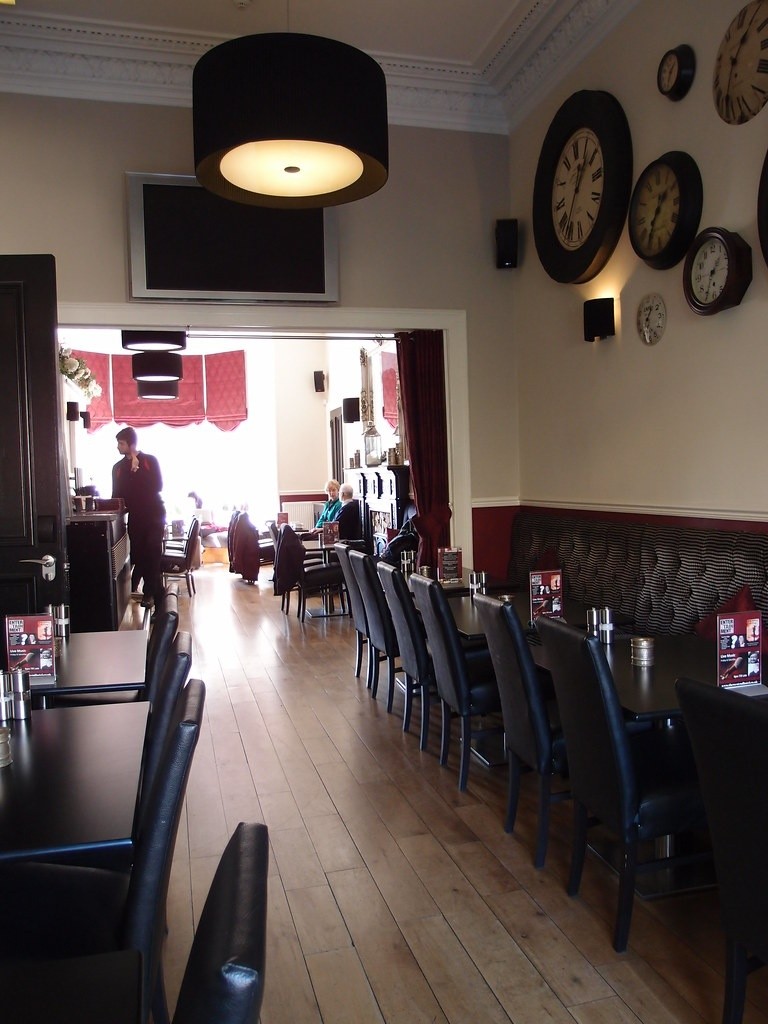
[282,501,314,532]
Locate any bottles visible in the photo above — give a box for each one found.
[599,607,614,645]
[468,570,480,601]
[498,595,514,603]
[586,607,600,638]
[56,604,70,637]
[401,551,410,572]
[46,603,57,636]
[0,669,12,721]
[480,571,487,596]
[55,637,62,658]
[420,566,431,577]
[409,551,416,572]
[10,670,32,720]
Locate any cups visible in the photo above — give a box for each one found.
[74,498,82,512]
[630,638,653,666]
[86,498,93,512]
[0,728,13,768]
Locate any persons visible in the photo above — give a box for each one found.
[748,651,759,676]
[309,479,362,541]
[111,426,166,623]
[40,648,53,670]
[552,597,561,614]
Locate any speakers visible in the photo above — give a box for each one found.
[315,371,324,392]
[495,219,518,268]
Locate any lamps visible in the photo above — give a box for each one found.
[192,1,389,209]
[342,397,360,424]
[363,425,382,467]
[584,298,615,342]
[120,330,187,401]
[360,347,380,436]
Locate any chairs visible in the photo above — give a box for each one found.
[0,511,768,1024]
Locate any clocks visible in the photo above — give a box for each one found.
[533,0,768,344]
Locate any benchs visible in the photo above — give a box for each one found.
[509,511,768,669]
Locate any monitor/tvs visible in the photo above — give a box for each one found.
[122,170,339,302]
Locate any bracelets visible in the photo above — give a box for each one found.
[133,466,139,471]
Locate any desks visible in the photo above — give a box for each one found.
[530,638,716,902]
[301,540,352,619]
[379,565,510,696]
[30,630,148,709]
[1,700,150,862]
[412,591,634,768]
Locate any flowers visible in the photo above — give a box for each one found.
[59,345,103,398]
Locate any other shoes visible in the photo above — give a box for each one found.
[141,592,155,608]
[150,605,162,623]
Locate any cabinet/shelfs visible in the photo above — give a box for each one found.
[342,466,411,556]
